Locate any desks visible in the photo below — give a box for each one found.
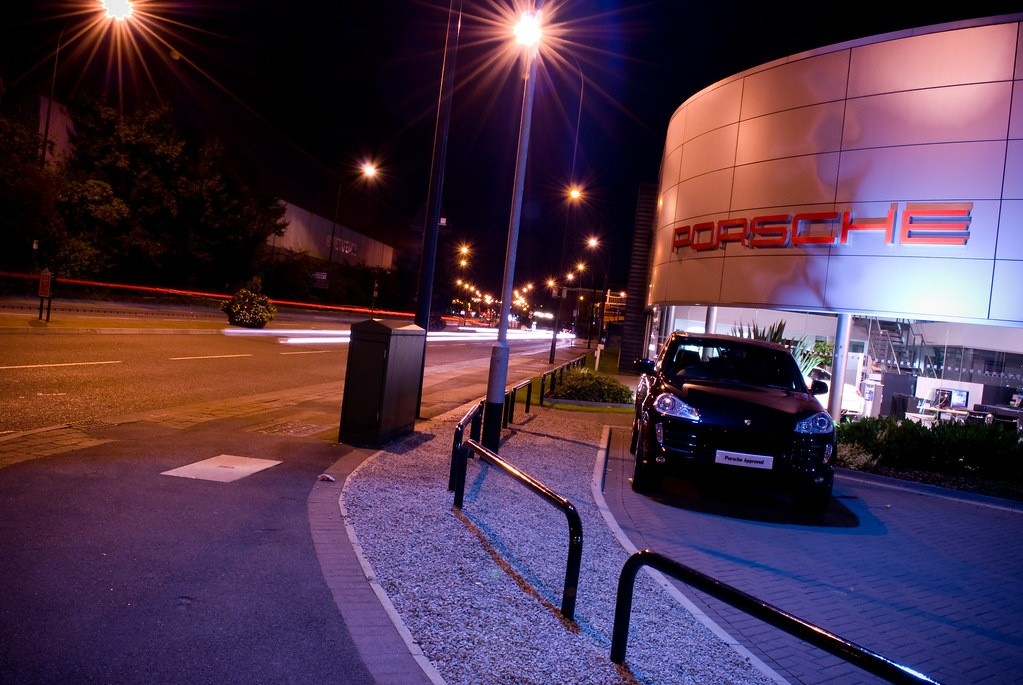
[917,406,969,420]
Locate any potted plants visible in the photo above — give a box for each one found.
[803,340,834,379]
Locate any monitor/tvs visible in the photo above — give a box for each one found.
[933,389,969,410]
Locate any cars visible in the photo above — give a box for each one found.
[628,328,839,509]
[798,364,867,416]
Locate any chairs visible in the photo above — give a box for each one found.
[663,349,704,376]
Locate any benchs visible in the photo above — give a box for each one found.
[906,412,935,430]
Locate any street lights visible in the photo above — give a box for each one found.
[478,11,549,463]
[39,5,614,366]
